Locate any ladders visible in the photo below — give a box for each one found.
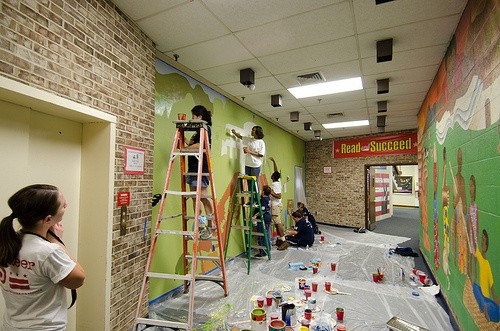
[131,119,229,331]
[223,175,272,275]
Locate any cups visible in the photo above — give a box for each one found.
[321,235,325,240]
[266,297,272,306]
[257,299,264,308]
[275,297,282,309]
[299,279,306,290]
[304,309,312,324]
[313,268,318,274]
[304,286,310,297]
[378,275,383,281]
[269,319,285,331]
[331,262,336,271]
[177,114,187,120]
[306,291,311,300]
[373,274,379,282]
[312,282,317,292]
[251,308,266,331]
[420,275,426,284]
[336,308,344,320]
[325,282,331,291]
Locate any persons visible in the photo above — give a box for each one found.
[181,105,217,240]
[285,202,318,248]
[250,185,273,258]
[232,126,266,207]
[0,184,86,331]
[269,156,289,251]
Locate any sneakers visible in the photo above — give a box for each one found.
[243,201,258,207]
[254,253,267,258]
[198,225,206,234]
[200,227,216,239]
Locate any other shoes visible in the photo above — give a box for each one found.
[277,241,289,250]
[276,237,283,246]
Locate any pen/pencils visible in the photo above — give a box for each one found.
[378,267,385,275]
